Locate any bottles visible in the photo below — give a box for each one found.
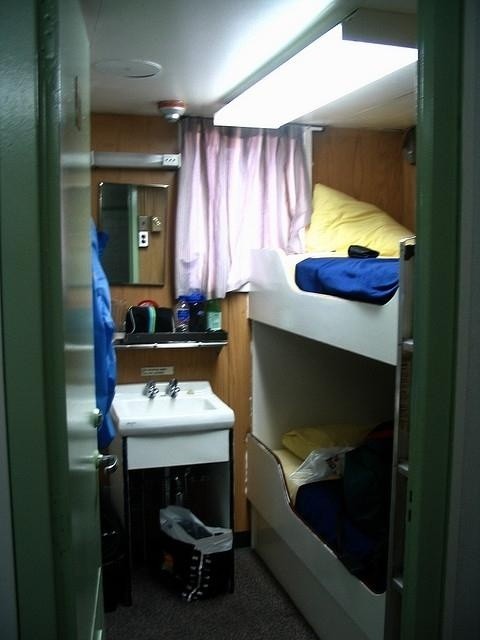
[191,288,204,333]
[174,293,191,333]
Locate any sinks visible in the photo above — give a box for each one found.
[110,380,235,470]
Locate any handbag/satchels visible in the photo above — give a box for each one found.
[124,298,172,333]
[157,505,235,603]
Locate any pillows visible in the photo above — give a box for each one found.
[307,183,416,255]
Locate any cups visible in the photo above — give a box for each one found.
[110,297,129,334]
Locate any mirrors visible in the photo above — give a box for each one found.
[96,180,169,287]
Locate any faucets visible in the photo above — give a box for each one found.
[165,377,181,399]
[141,379,159,399]
